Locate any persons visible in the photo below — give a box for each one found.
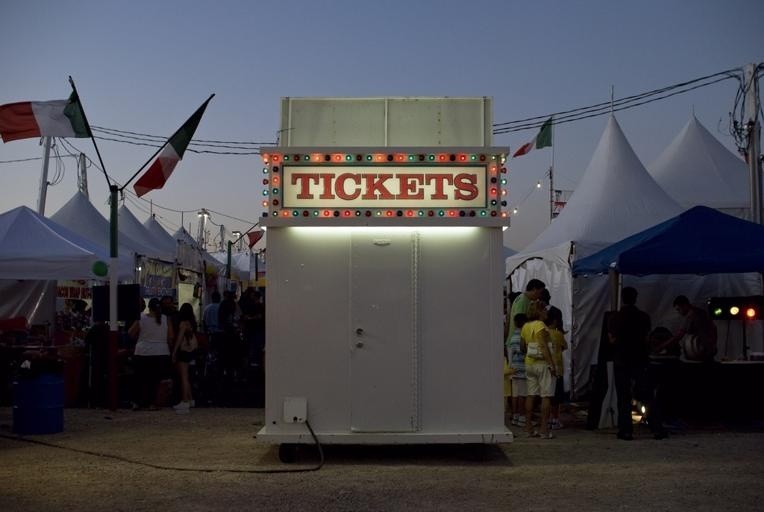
[664,295,718,362]
[84,286,265,415]
[608,286,670,441]
[502,278,568,439]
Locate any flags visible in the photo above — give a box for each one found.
[133,100,208,198]
[0,92,91,143]
[512,117,552,158]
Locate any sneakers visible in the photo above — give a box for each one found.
[173,400,194,410]
[511,415,565,438]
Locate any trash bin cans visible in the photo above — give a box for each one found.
[11,359,65,434]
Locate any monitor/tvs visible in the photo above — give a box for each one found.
[139,255,175,298]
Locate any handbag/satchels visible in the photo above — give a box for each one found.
[526,343,555,358]
[180,333,199,352]
